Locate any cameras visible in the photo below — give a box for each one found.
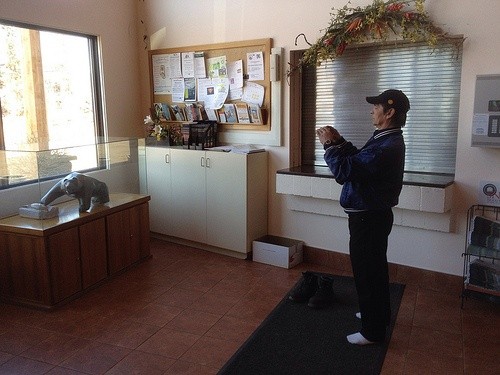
[326,126,334,133]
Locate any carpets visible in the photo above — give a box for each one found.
[214,271,407,375]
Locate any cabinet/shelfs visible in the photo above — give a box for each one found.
[0,195,154,312]
[458,202,500,312]
[138,137,269,260]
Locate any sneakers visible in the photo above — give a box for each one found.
[307,274,336,308]
[289,271,320,303]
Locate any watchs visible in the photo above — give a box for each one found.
[323,140,335,150]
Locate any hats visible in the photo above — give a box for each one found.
[366,90,410,113]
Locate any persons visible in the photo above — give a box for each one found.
[316,89,410,345]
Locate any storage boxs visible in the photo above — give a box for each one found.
[251,235,304,269]
[0,135,147,230]
